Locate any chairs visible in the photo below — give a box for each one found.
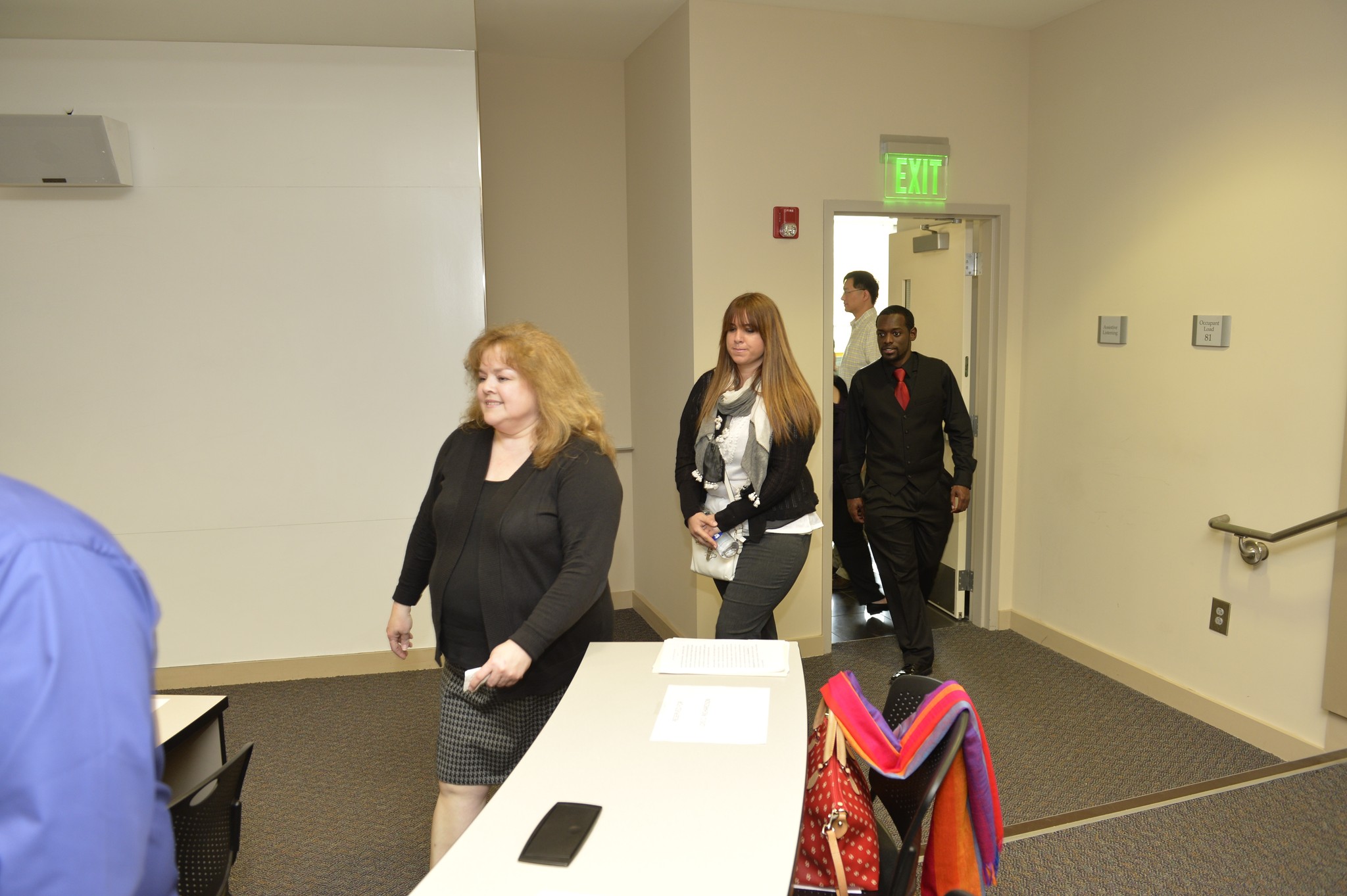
[166,741,253,896]
[787,674,970,896]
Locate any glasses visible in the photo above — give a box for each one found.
[844,288,865,296]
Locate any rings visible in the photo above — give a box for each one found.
[702,527,705,529]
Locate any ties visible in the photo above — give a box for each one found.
[894,368,910,411]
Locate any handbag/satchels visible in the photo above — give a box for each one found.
[690,470,746,581]
[795,696,880,891]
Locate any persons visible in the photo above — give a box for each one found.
[0,473,184,895]
[831,271,883,590]
[673,293,825,640]
[841,305,978,683]
[832,340,891,614]
[386,323,624,875]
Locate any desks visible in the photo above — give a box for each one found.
[149,690,228,802]
[420,638,808,896]
